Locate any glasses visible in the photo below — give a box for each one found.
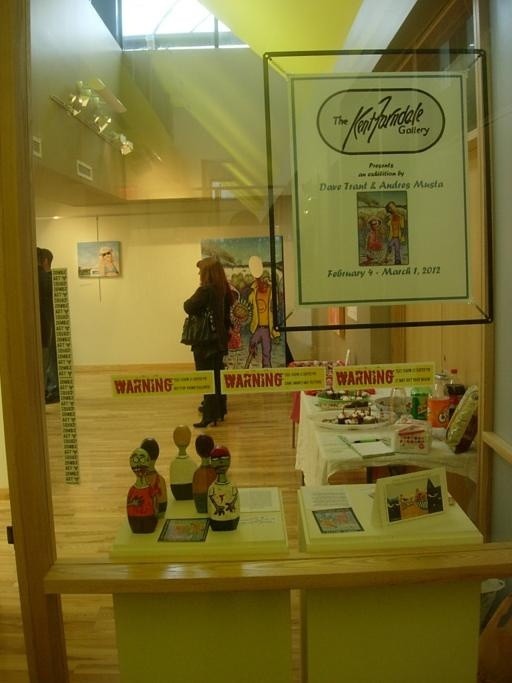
[103,252,110,256]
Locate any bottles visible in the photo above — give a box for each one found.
[389,367,465,436]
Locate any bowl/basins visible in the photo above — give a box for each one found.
[312,391,372,409]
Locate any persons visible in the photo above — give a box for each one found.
[35,247,60,405]
[98,247,120,277]
[364,201,405,265]
[183,257,227,428]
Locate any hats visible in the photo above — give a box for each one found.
[367,215,382,229]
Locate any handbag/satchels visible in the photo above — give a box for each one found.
[180,307,217,346]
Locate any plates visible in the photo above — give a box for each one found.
[307,411,391,430]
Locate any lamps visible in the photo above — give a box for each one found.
[50,94,133,156]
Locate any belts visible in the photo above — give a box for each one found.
[258,325,267,329]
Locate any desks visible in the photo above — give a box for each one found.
[295,388,481,487]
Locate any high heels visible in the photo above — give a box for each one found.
[193,416,217,428]
[218,413,224,421]
